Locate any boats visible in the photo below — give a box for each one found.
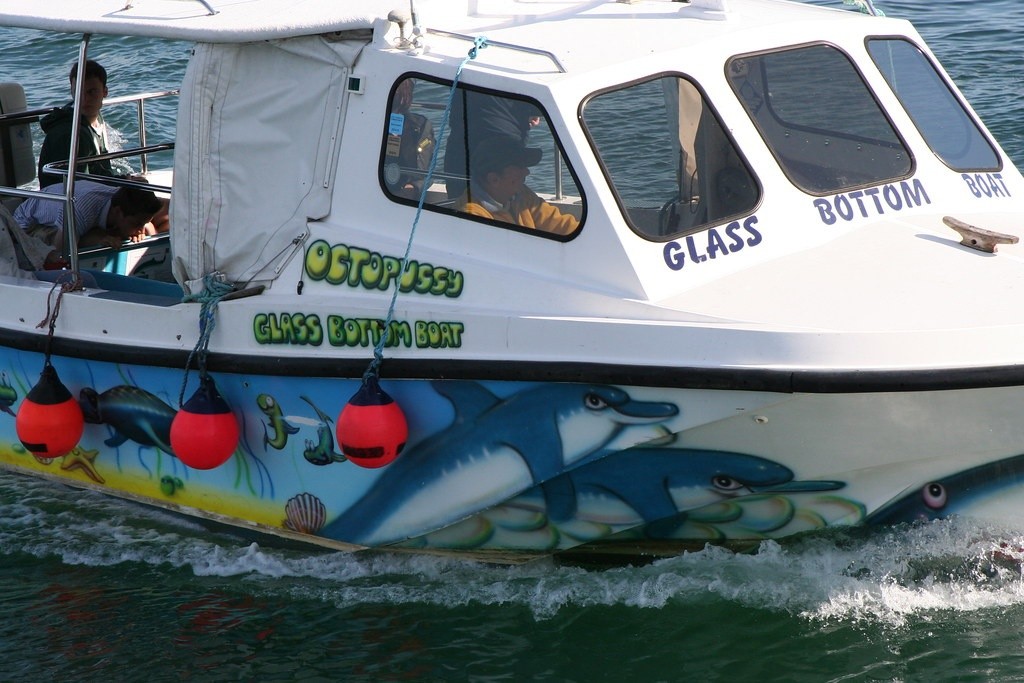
[0,0,1024,569]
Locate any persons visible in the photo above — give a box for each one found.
[13,59,170,251]
[384,78,581,243]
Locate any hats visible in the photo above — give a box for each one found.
[473,135,543,167]
